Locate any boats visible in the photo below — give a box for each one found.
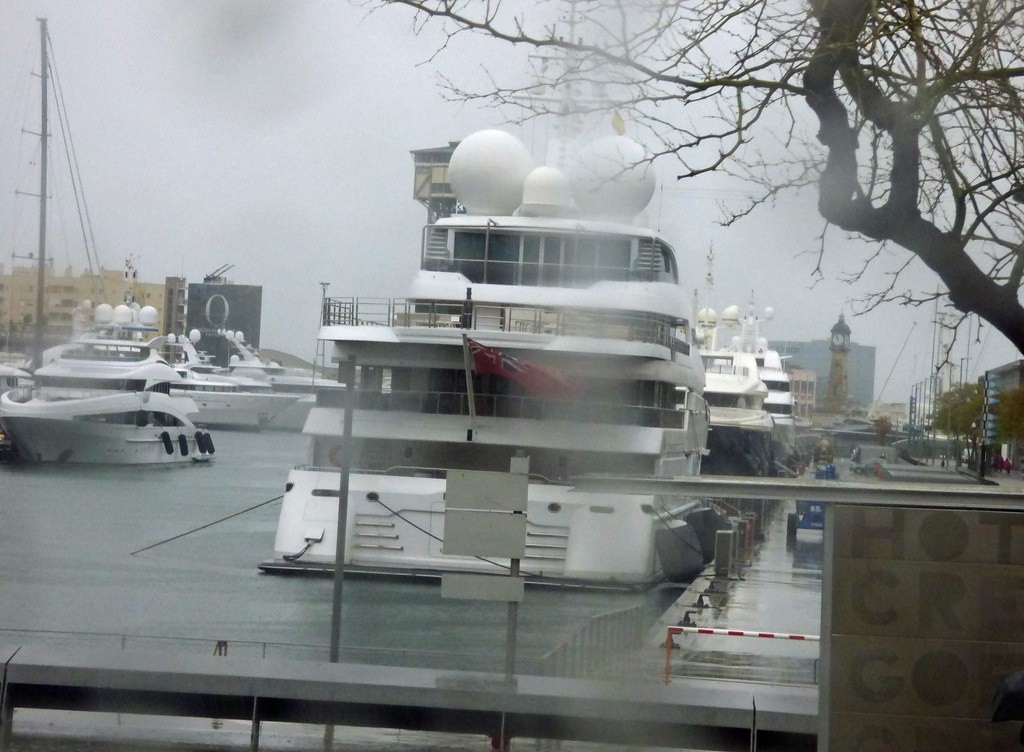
[159,330,298,429]
[742,306,794,437]
[259,127,703,593]
[2,303,213,465]
[235,330,347,432]
[690,304,776,471]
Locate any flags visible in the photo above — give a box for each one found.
[468,337,577,402]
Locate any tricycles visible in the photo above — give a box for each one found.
[786,460,848,538]
